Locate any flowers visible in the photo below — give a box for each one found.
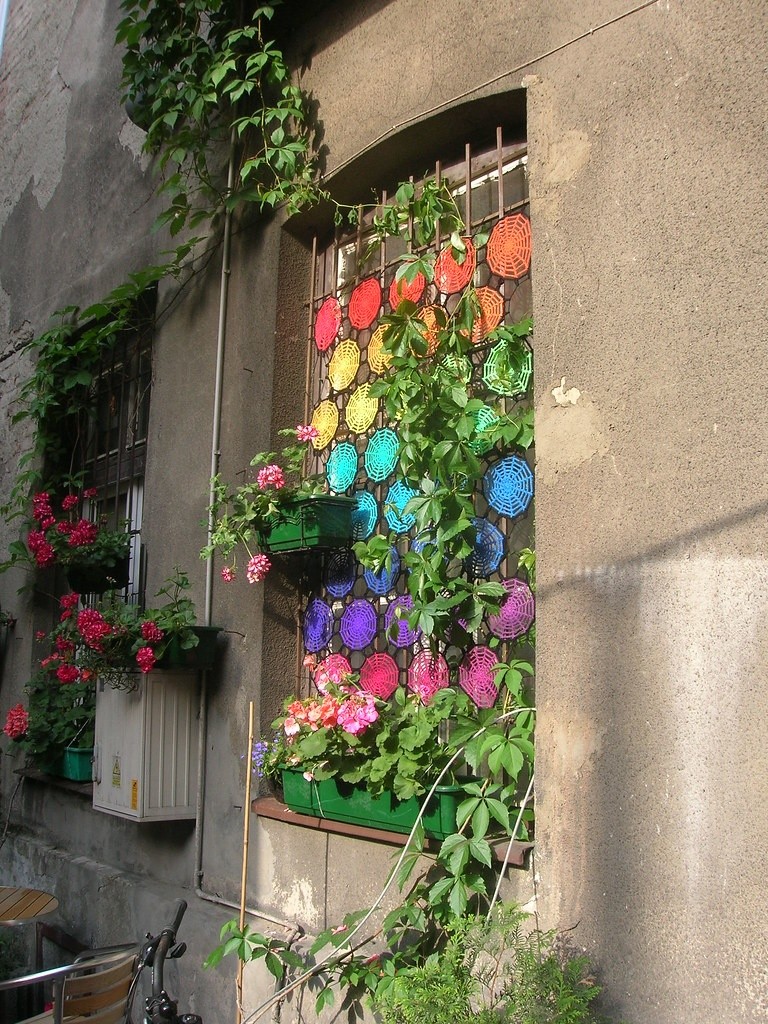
[199,424,320,584]
[33,566,199,694]
[240,674,468,803]
[0,485,135,602]
[2,666,99,754]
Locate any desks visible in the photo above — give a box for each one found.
[0,885,61,1019]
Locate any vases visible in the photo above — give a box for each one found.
[52,741,93,781]
[252,493,357,551]
[106,625,225,674]
[64,553,129,594]
[279,757,491,840]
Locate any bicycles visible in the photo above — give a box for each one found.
[123,898,206,1024]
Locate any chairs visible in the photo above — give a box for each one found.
[0,943,138,1024]
